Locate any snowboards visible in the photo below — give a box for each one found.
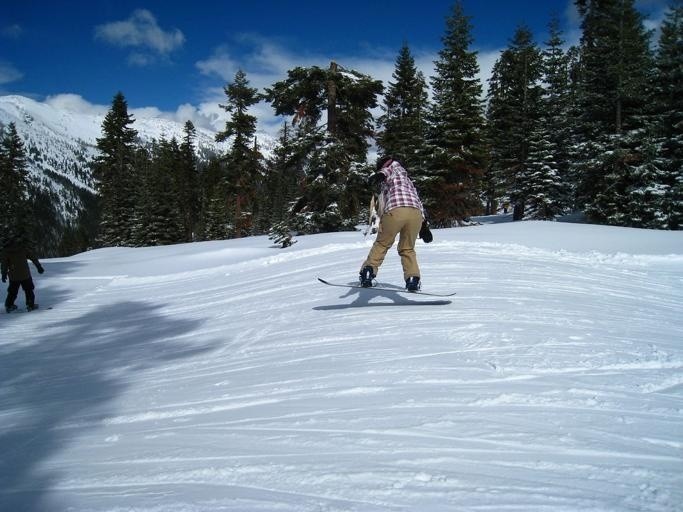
[1,307,52,313]
[318,277,457,297]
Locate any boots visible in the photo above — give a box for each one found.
[405,277,421,292]
[360,267,377,287]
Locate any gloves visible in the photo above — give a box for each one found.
[369,173,385,185]
[420,221,434,243]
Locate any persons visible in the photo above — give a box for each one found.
[0,236,44,313]
[359,157,433,292]
